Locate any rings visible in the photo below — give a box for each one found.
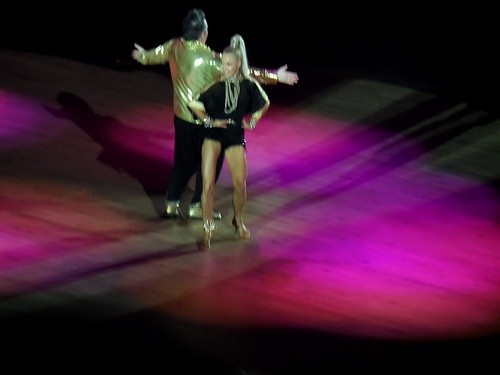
[228,118,232,124]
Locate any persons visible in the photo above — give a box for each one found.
[187,32,271,254]
[132,8,299,219]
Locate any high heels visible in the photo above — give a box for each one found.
[232,216,251,239]
[203,219,215,249]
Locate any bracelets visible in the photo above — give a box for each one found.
[203,113,214,128]
[249,118,256,131]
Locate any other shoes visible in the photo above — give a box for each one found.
[165,197,179,215]
[189,202,220,219]
